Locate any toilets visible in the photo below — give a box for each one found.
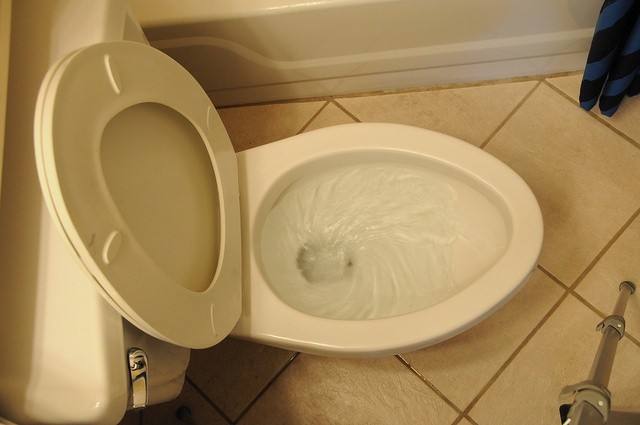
[0,0,545,425]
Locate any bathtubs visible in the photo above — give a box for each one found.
[127,2,602,108]
